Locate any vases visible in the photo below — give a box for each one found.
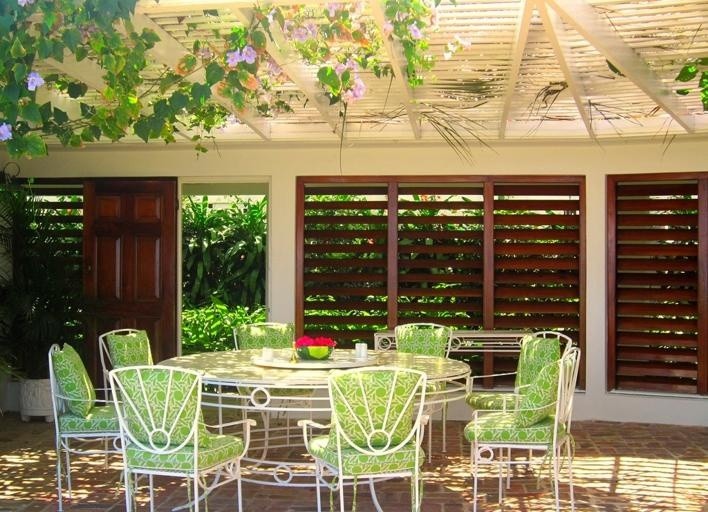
[295,345,332,360]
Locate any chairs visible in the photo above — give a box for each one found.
[230,321,317,451]
[99,328,154,470]
[296,365,429,511]
[48,343,129,511]
[464,329,571,490]
[395,322,453,462]
[109,364,257,512]
[464,347,582,512]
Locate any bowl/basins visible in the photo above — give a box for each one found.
[296,346,335,361]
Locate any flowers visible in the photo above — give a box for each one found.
[293,335,337,350]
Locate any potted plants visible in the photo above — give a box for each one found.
[0,162,86,424]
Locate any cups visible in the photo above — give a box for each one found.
[262,348,274,363]
[355,343,368,358]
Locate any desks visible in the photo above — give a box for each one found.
[372,328,532,392]
